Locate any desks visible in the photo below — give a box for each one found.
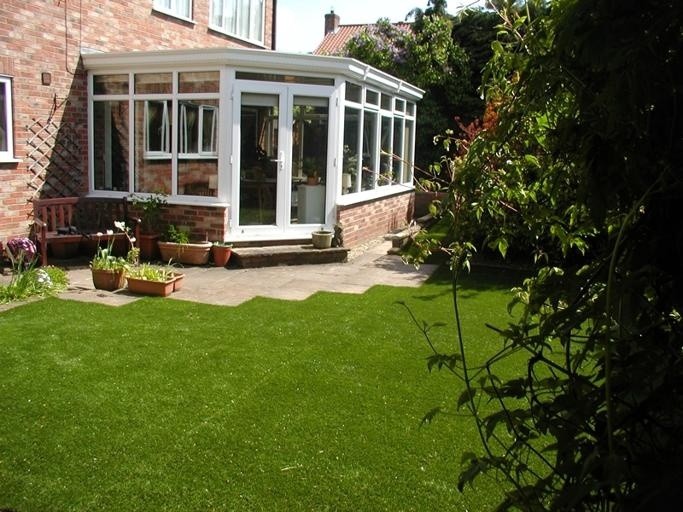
[297,185,325,225]
[240,177,277,224]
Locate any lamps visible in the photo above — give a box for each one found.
[341,174,352,196]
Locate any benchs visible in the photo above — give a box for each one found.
[31,197,142,266]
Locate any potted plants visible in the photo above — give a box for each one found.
[134,185,233,268]
[89,234,185,296]
[302,155,322,185]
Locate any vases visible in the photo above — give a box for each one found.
[312,230,332,248]
